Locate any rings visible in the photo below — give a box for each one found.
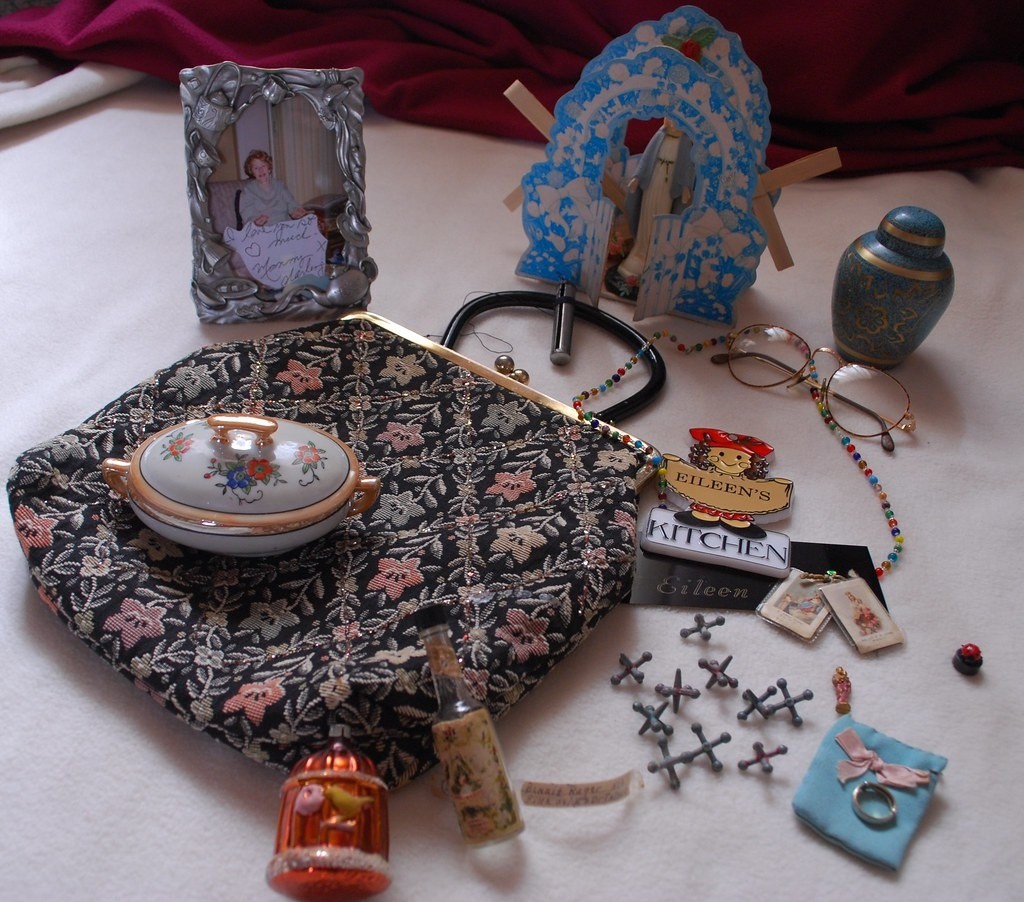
[851,781,896,824]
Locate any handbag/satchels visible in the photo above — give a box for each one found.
[9,294,668,801]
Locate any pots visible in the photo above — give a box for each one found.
[103,415,379,559]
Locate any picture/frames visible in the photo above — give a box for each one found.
[179,61,378,326]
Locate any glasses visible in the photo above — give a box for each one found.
[712,324,916,455]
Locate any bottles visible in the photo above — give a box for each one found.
[832,205,956,372]
[408,605,525,849]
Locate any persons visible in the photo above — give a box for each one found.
[618,118,696,287]
[237,152,316,227]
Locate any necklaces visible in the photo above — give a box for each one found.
[573,327,903,584]
